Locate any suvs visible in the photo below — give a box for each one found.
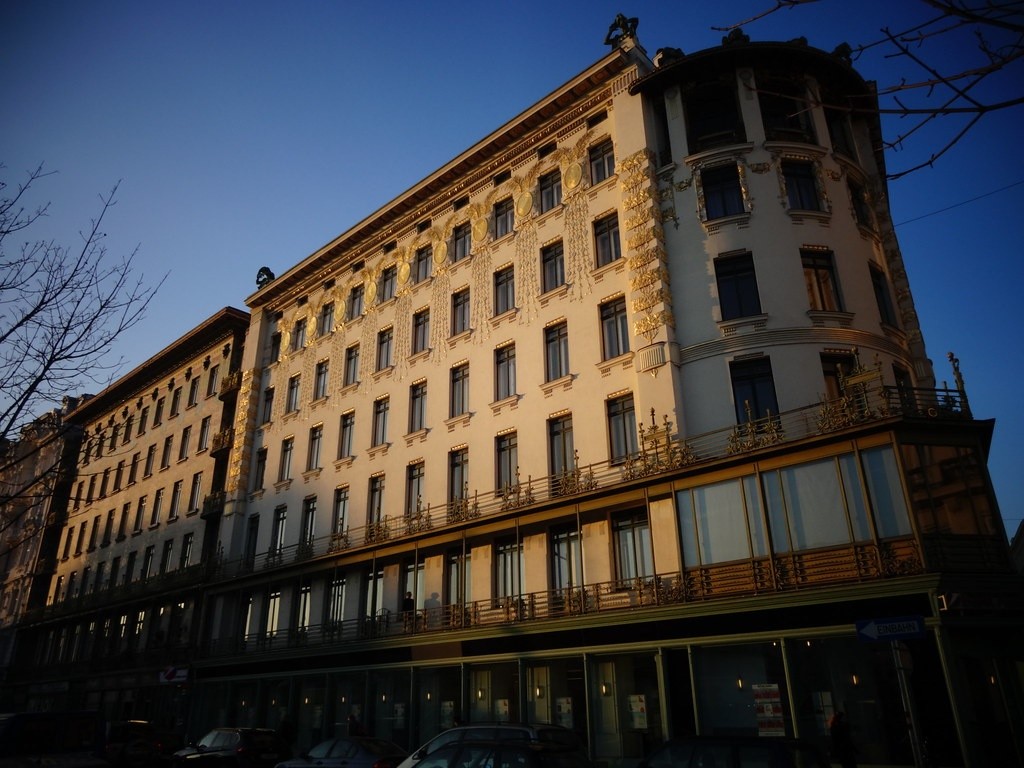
[103,721,163,768]
[170,724,278,768]
[399,719,577,768]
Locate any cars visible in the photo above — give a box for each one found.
[274,735,410,768]
[410,739,592,768]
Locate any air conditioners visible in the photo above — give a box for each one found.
[213,428,233,449]
[220,371,239,393]
[202,491,222,512]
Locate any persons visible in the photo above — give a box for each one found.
[348,714,369,737]
[830,710,861,768]
[400,592,414,612]
